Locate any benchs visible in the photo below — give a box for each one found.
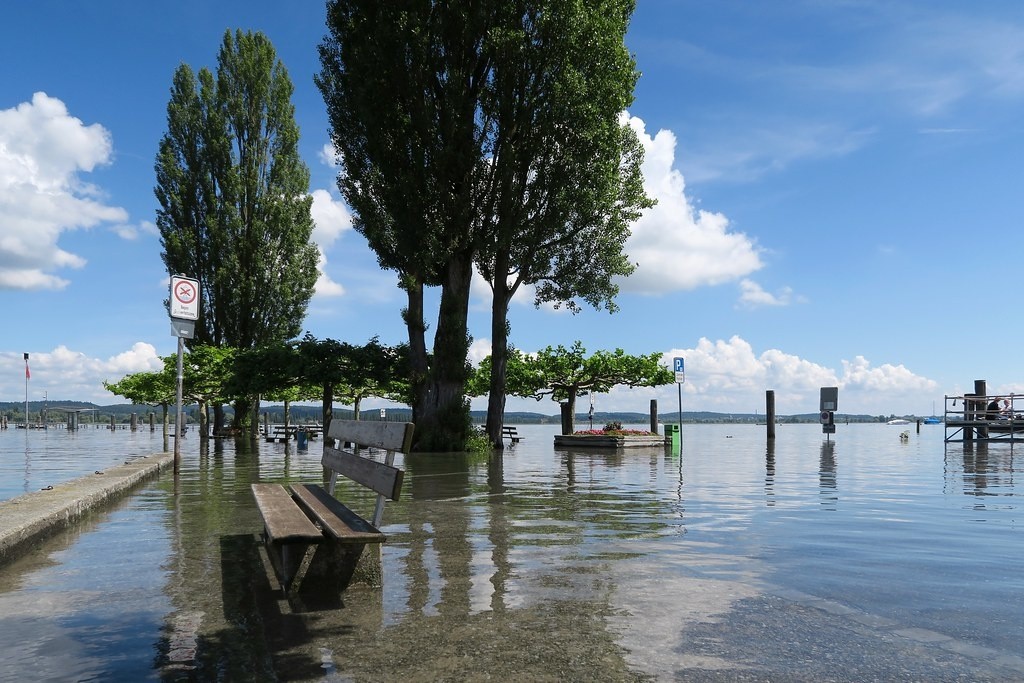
[481,424,525,443]
[266,426,296,443]
[300,424,324,441]
[170,426,190,437]
[250,420,416,599]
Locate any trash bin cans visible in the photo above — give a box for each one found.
[296,431,308,448]
[664,424,679,446]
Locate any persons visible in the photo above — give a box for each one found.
[1000,399,1012,419]
[985,397,1011,421]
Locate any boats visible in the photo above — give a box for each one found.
[924,416,941,424]
[887,418,911,426]
